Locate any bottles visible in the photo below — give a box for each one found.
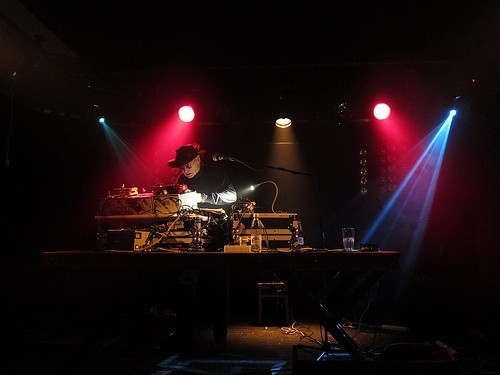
[250,213,262,252]
[298,228,304,245]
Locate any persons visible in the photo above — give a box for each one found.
[166,144,259,215]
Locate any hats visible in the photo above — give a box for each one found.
[168,144,203,168]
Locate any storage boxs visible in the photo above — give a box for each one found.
[231,212,297,248]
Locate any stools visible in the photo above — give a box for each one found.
[257,279,290,324]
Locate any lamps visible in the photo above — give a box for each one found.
[251,180,285,214]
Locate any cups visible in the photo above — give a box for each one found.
[342,227,355,252]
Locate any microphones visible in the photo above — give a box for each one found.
[212,154,235,162]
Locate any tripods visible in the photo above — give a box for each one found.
[296,321,347,362]
